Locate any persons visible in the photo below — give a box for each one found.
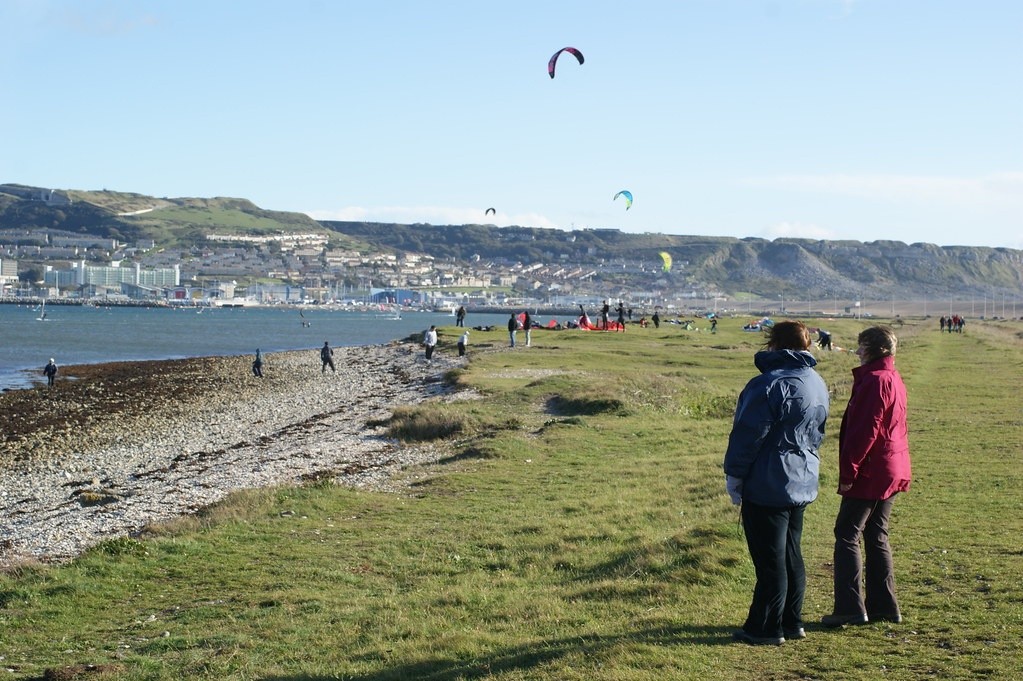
[457,331,469,356]
[615,303,626,332]
[628,308,632,319]
[940,315,966,333]
[522,311,531,346]
[642,317,648,327]
[723,321,830,645]
[651,312,660,328]
[709,317,718,331]
[600,300,609,332]
[578,305,588,328]
[320,341,335,373]
[819,326,911,624]
[423,325,438,359]
[456,306,466,327]
[508,312,517,347]
[43,358,57,386]
[252,348,264,377]
[814,328,831,351]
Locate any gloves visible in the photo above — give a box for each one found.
[726,475,743,506]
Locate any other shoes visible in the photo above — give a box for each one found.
[866,611,902,624]
[732,630,785,645]
[783,627,806,639]
[822,612,869,628]
